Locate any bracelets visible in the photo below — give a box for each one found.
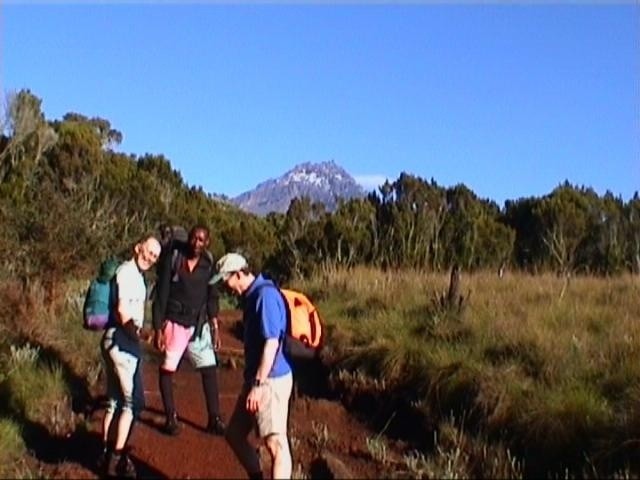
[253,378,260,386]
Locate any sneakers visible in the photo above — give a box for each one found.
[205,414,228,436]
[163,417,183,435]
[95,448,138,478]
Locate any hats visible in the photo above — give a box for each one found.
[208,252,250,286]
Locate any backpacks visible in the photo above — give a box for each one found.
[159,223,214,282]
[278,286,324,362]
[81,260,118,330]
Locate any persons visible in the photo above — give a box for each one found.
[96,232,163,480]
[151,223,229,436]
[206,252,294,480]
